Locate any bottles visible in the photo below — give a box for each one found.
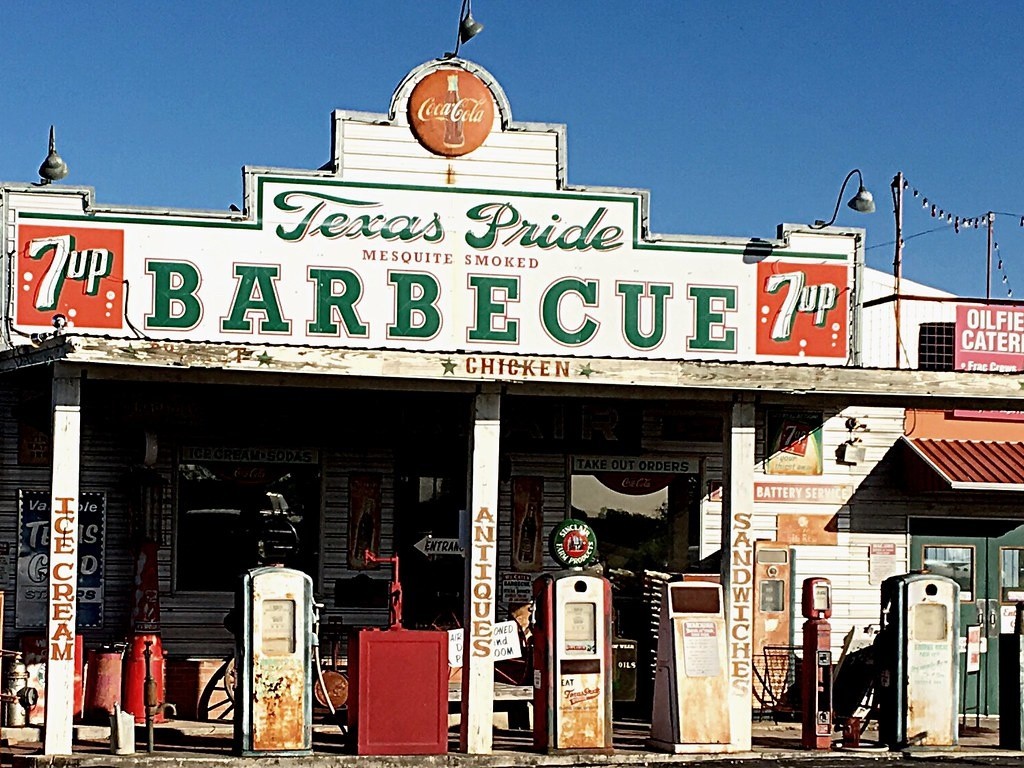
[443,73,465,148]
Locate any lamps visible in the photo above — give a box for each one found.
[823,167,876,228]
[450,0,483,57]
[36,124,69,184]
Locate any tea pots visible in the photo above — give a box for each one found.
[109,701,136,755]
